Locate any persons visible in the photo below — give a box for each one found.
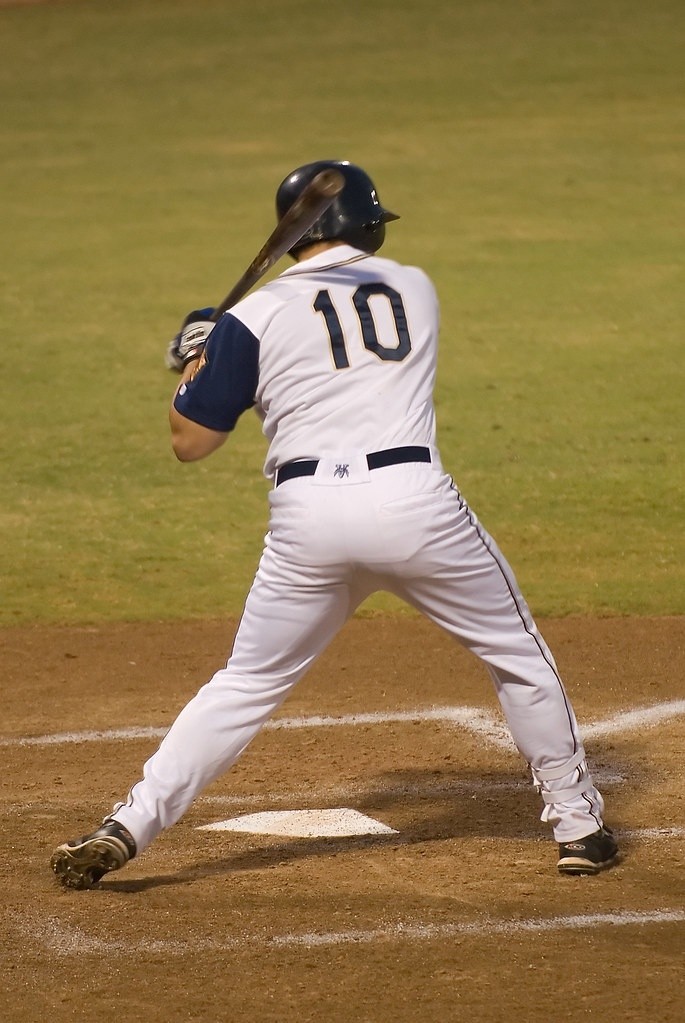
[52,158,621,888]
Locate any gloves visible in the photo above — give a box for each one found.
[165,307,216,371]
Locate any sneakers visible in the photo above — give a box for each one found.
[51,819,136,890]
[557,826,618,873]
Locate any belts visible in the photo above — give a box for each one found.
[277,446,430,486]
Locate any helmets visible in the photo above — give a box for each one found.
[275,159,401,254]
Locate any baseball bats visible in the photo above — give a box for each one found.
[206,168,342,325]
